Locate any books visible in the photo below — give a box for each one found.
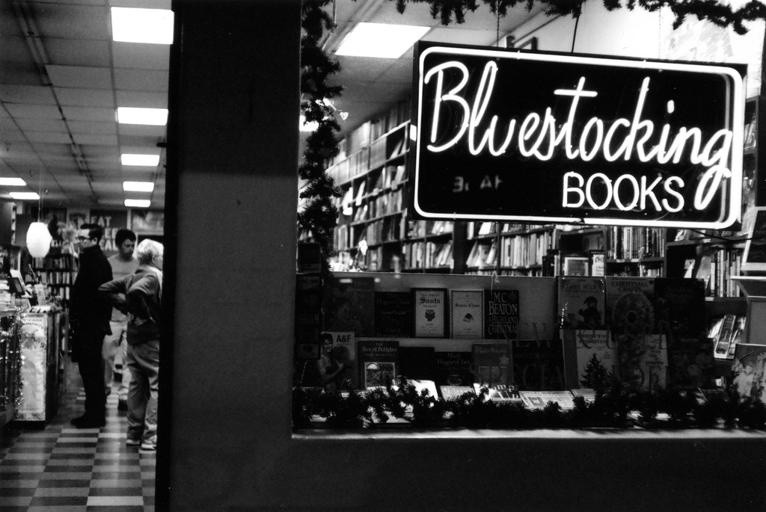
[298,276,746,357]
[297,136,455,271]
[465,172,746,297]
[0,236,88,301]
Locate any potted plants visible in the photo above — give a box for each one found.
[297,35,345,271]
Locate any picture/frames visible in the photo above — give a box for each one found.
[411,286,486,341]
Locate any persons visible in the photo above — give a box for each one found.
[67,223,166,451]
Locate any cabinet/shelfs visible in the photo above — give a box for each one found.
[297,96,765,304]
[21,255,79,304]
[19,307,67,420]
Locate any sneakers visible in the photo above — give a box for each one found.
[71,417,106,428]
[126,430,157,449]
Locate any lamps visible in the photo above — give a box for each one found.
[26,130,53,260]
